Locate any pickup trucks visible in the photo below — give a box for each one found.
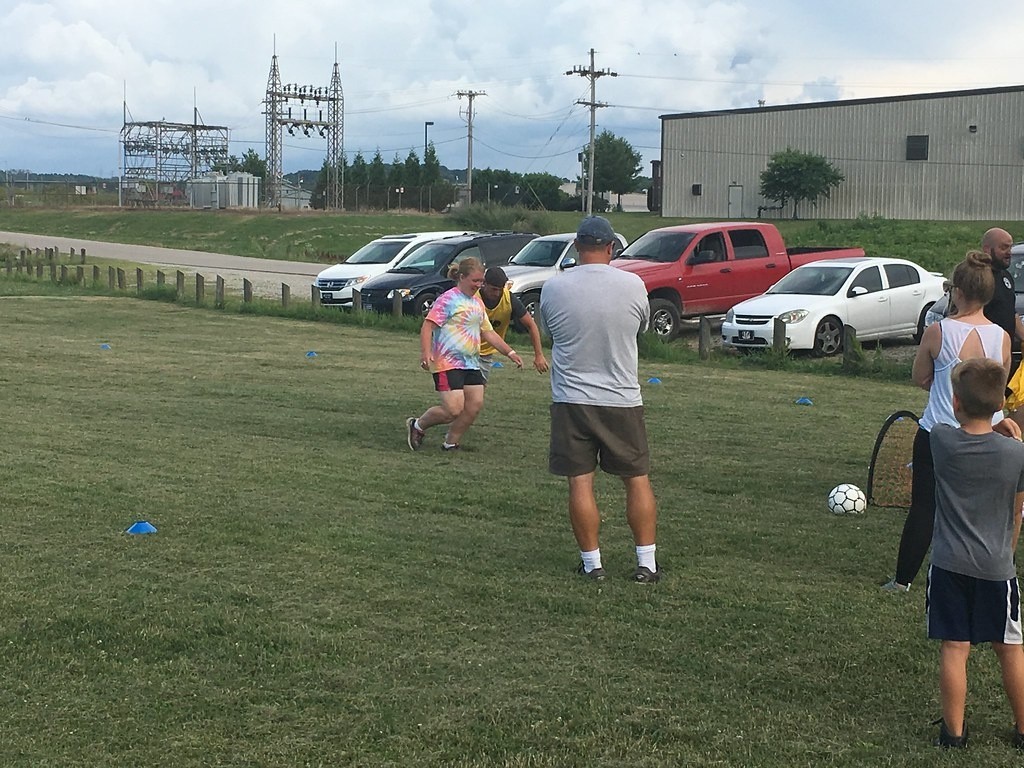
[603,220,867,347]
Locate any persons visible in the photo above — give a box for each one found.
[925,358,1024,750]
[1002,359,1024,421]
[540,216,662,585]
[406,258,523,451]
[983,228,1024,401]
[445,267,549,439]
[879,250,1012,592]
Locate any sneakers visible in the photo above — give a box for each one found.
[440,442,460,450]
[405,417,425,452]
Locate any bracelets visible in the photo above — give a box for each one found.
[507,350,517,357]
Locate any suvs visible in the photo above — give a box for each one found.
[357,230,544,321]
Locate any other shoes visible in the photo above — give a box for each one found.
[879,581,908,592]
[1014,723,1024,750]
[635,560,662,585]
[576,561,605,581]
[932,717,969,749]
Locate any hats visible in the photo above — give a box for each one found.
[484,266,507,288]
[577,216,613,244]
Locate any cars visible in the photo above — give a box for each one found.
[924,243,1024,362]
[719,256,954,358]
[480,231,628,331]
[310,229,480,313]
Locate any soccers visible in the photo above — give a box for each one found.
[827,482,867,515]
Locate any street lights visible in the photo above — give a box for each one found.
[425,121,435,166]
